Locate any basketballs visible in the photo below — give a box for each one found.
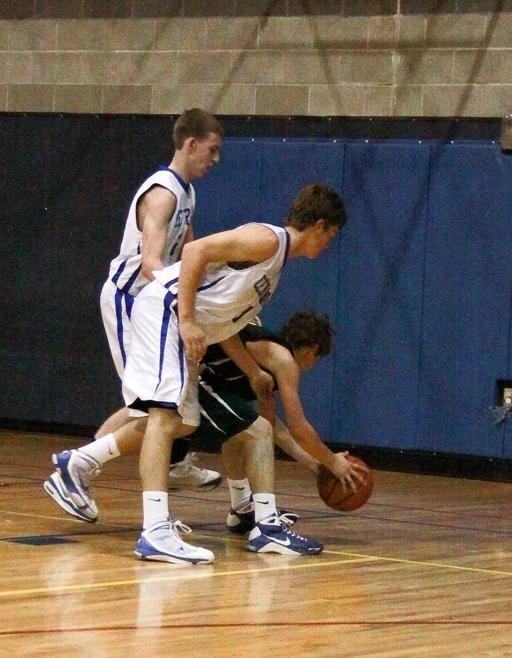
[316,455,374,511]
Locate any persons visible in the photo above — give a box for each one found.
[93,108,223,489]
[52,181,347,563]
[44,306,369,559]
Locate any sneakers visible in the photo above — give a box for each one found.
[134,520,215,565]
[43,449,99,521]
[169,453,221,492]
[226,506,324,555]
